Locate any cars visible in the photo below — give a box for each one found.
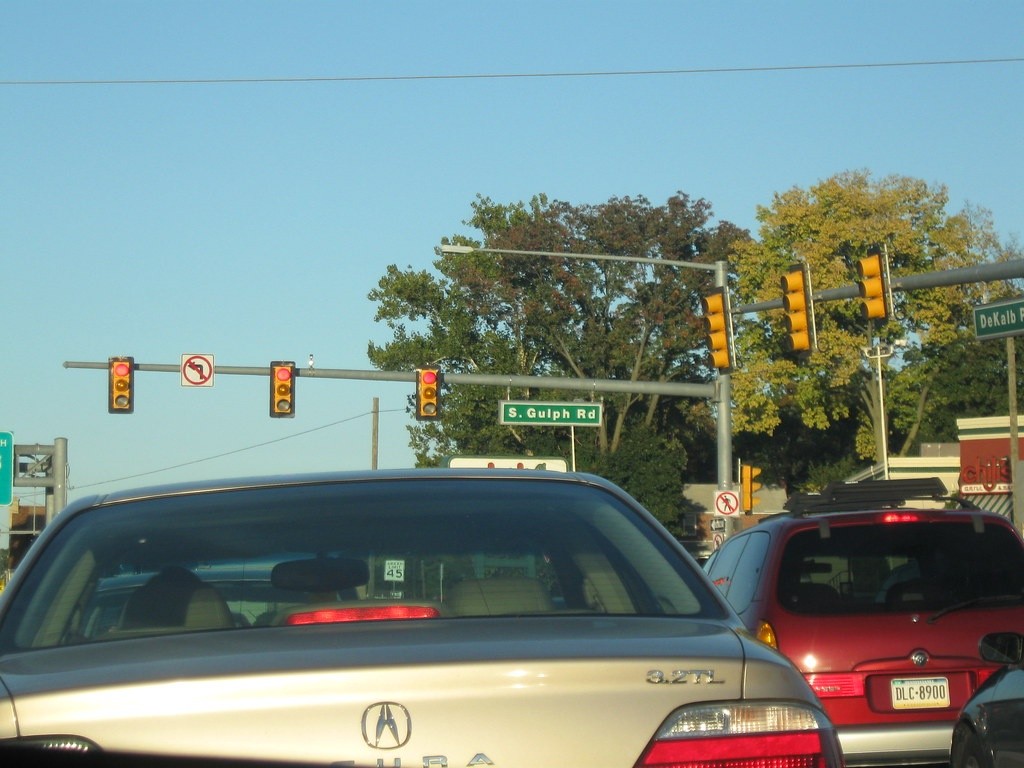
[948,627,1022,767]
[0,461,842,768]
[683,493,1022,767]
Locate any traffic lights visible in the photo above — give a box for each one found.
[108,355,133,411]
[417,365,441,419]
[781,262,815,351]
[855,241,893,322]
[270,361,294,417]
[700,284,735,371]
